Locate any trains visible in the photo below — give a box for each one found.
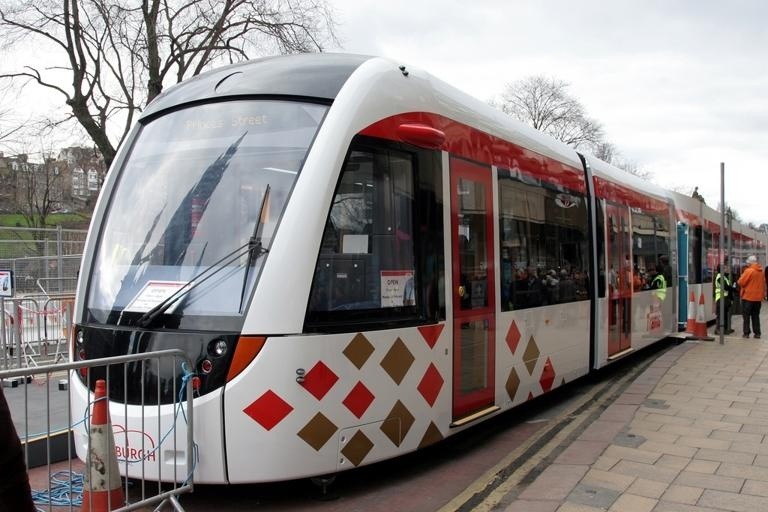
[67,50,768,497]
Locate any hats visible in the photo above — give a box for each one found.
[746,256,756,264]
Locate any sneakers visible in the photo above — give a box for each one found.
[715,328,733,335]
[743,334,749,338]
[754,335,760,338]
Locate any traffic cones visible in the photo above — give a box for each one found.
[80,377,125,512]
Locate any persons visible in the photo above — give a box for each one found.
[715,262,735,334]
[736,255,766,338]
[0,384,36,512]
[506,259,668,306]
